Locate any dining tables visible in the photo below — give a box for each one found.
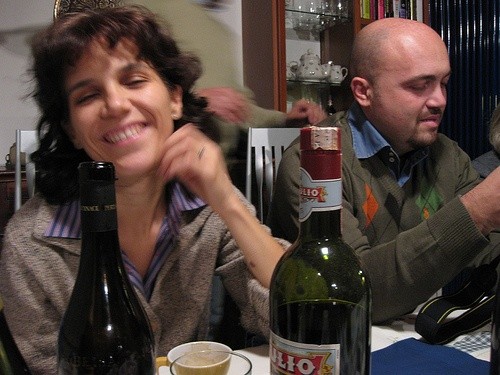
[228,288,491,375]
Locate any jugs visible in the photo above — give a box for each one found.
[286,49,349,85]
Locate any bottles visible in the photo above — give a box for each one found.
[58,161,156,375]
[270,126,372,375]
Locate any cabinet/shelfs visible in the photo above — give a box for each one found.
[242,1,429,113]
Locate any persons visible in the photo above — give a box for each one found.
[265,18,500,322]
[115,0,326,152]
[471,104,500,180]
[1,5,293,374]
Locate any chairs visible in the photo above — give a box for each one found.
[245,127,302,225]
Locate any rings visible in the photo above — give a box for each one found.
[198,147,206,160]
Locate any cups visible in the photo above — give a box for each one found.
[170,350,253,375]
[167,340,232,375]
[284,0,348,31]
